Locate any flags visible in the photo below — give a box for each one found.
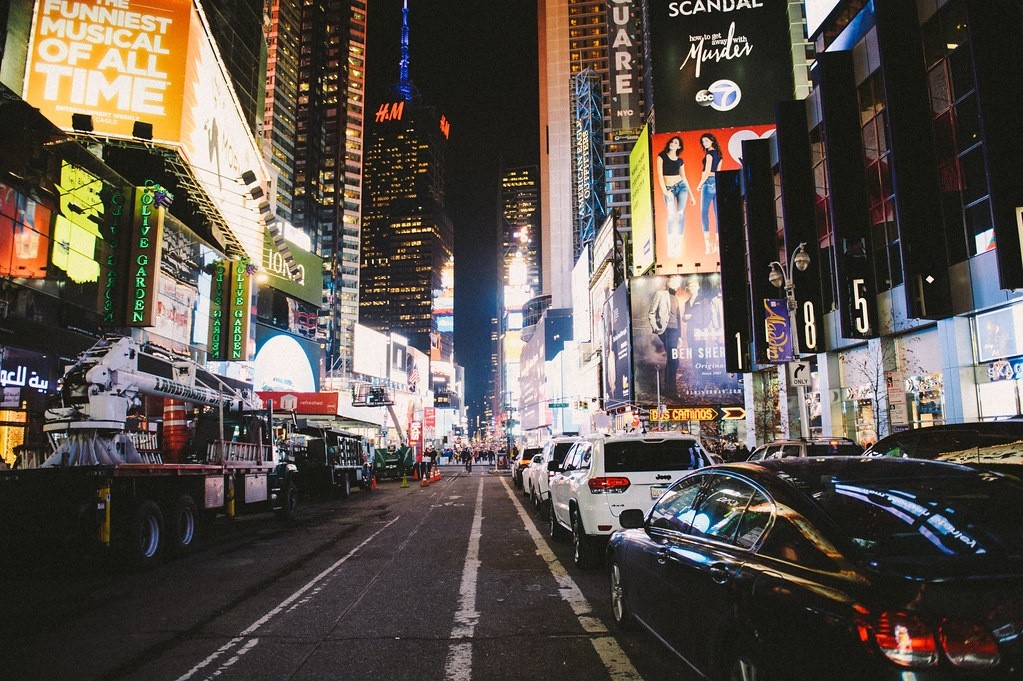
[409,364,416,384]
[416,366,420,384]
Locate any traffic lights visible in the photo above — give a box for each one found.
[578,401,583,408]
[583,401,588,410]
[574,401,578,410]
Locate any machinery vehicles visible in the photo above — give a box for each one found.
[0,332,301,579]
[349,379,416,481]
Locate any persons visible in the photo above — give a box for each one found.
[649,275,743,404]
[657,136,696,257]
[424,444,519,477]
[703,440,756,463]
[696,133,723,254]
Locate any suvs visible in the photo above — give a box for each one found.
[545,430,719,571]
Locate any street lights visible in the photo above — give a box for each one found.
[766,240,811,439]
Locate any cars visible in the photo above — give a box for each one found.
[861,414,1022,483]
[508,433,582,522]
[744,436,865,462]
[604,453,1022,681]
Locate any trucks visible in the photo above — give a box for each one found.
[280,410,374,500]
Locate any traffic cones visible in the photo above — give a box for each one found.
[371,475,377,491]
[399,472,410,489]
[413,468,419,482]
[428,468,442,483]
[419,472,429,487]
